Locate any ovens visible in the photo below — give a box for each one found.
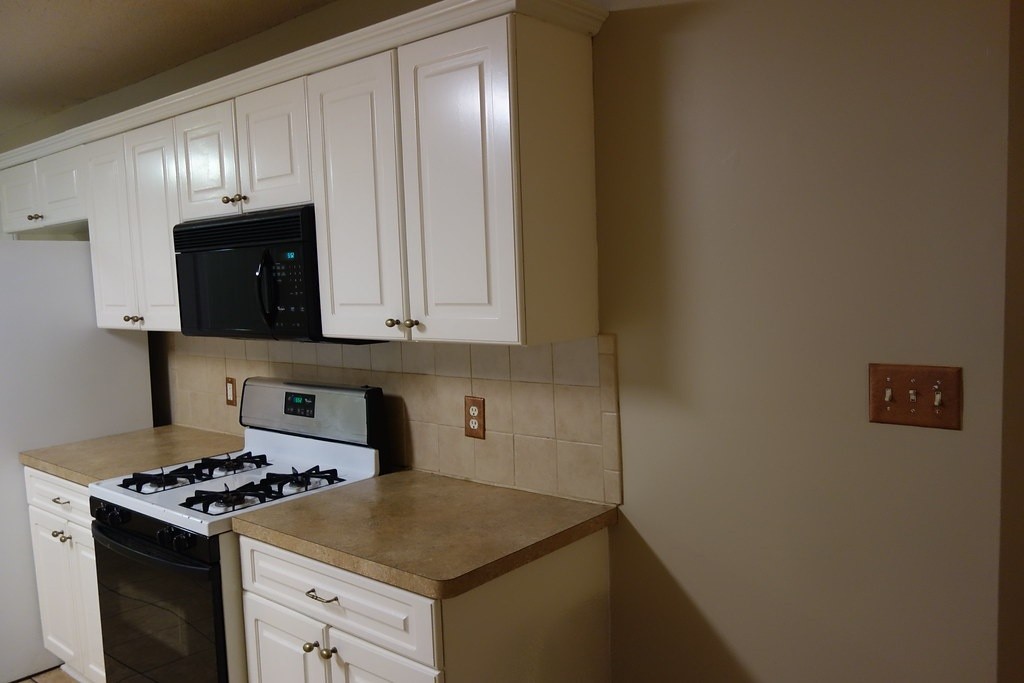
[89,496,248,683]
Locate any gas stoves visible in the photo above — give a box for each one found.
[88,376,405,538]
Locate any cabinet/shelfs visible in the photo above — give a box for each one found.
[233,469,619,683]
[0,0,610,348]
[19,423,244,683]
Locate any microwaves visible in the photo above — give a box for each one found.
[174,203,390,345]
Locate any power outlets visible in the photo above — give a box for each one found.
[464,396,485,440]
[225,377,238,406]
